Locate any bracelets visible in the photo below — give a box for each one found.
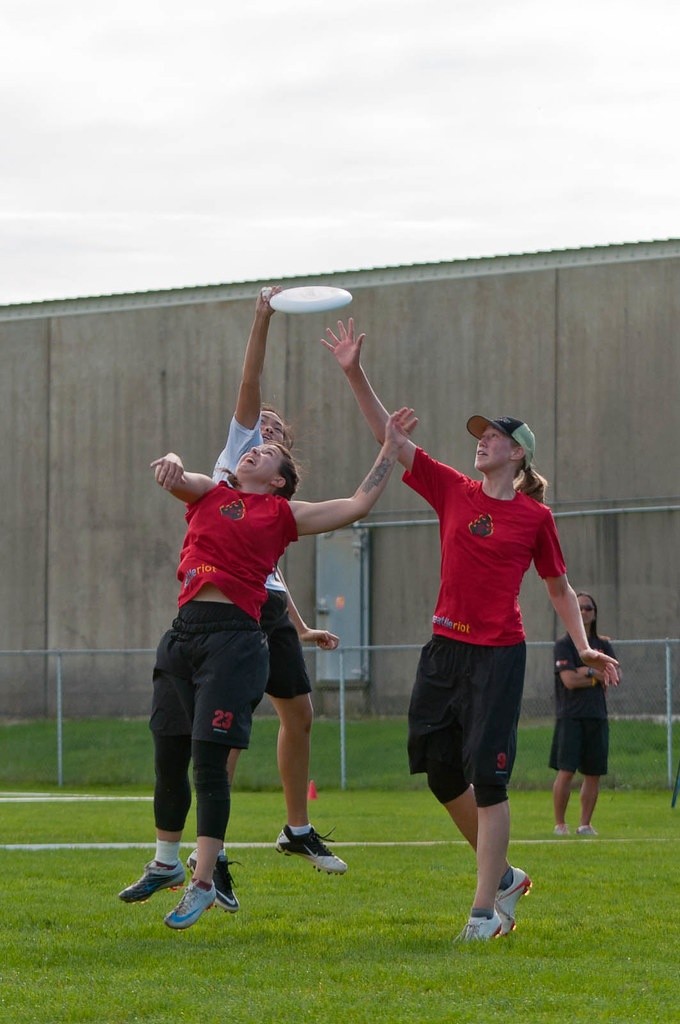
[588,667,594,678]
[592,677,596,686]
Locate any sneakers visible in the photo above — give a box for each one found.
[275,824,347,875]
[454,907,502,945]
[494,866,532,939]
[186,848,245,913]
[118,859,185,905]
[164,880,216,930]
[576,823,598,835]
[554,823,571,835]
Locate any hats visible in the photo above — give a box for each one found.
[466,415,535,470]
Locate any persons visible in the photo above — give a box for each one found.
[320,317,620,939]
[119,285,419,929]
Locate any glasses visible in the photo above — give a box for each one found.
[580,605,594,611]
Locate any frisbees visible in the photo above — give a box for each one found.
[269,286,353,315]
